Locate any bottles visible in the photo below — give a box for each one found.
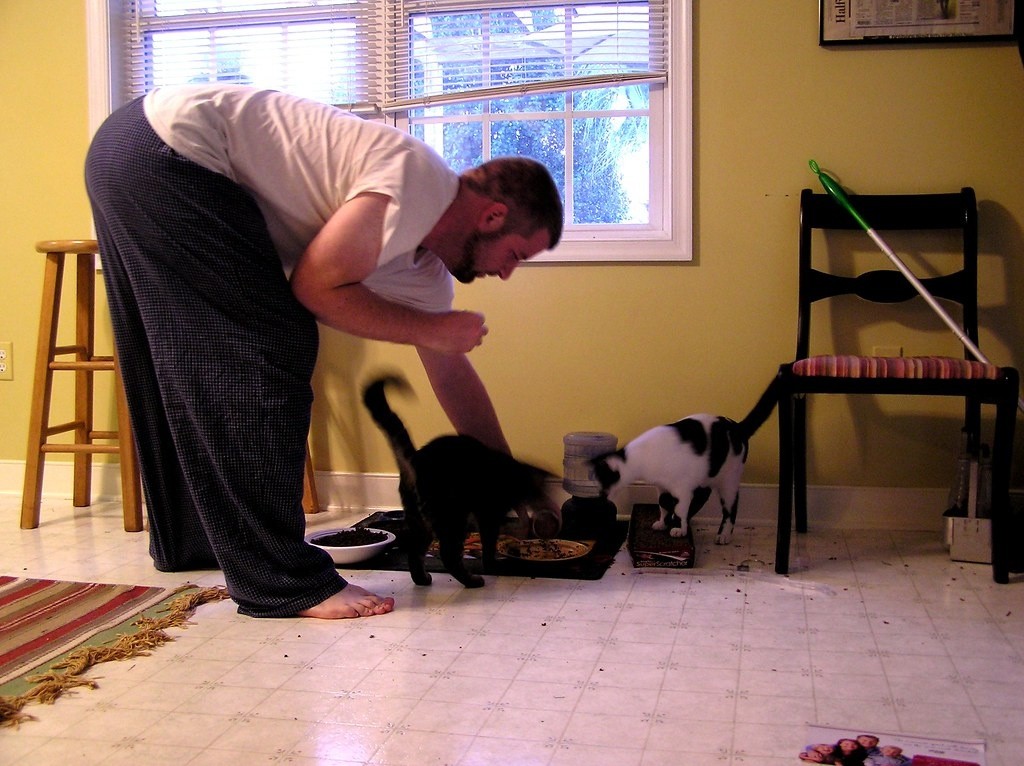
[562,431,620,498]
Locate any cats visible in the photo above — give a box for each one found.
[589,375,778,545]
[362,376,563,588]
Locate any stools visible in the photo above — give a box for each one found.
[19,237,150,532]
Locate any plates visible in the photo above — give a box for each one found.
[498,538,589,561]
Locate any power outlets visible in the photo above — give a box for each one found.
[0,341,13,382]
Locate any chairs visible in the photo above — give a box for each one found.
[774,187,1021,585]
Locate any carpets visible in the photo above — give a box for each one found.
[334,512,629,581]
[0,575,230,731]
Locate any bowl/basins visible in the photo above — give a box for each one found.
[303,527,396,563]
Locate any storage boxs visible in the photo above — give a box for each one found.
[630,501,695,569]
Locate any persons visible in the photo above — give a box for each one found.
[799,734,912,766]
[84,81,563,620]
